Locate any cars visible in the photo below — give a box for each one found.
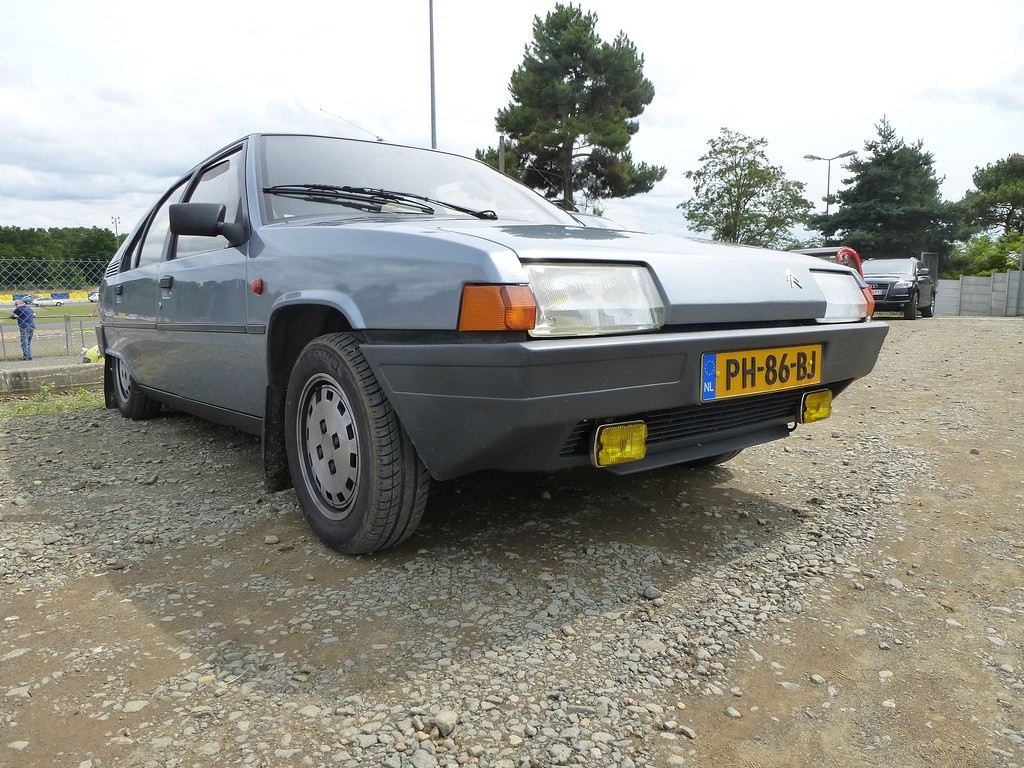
[95,131,888,559]
[82,345,105,364]
[21,294,36,304]
[88,292,98,304]
[30,297,65,307]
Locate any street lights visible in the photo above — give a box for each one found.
[802,150,858,247]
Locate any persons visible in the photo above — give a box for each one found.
[9,300,37,361]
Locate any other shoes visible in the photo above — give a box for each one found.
[23,357,32,361]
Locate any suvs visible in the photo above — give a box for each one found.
[859,256,937,322]
